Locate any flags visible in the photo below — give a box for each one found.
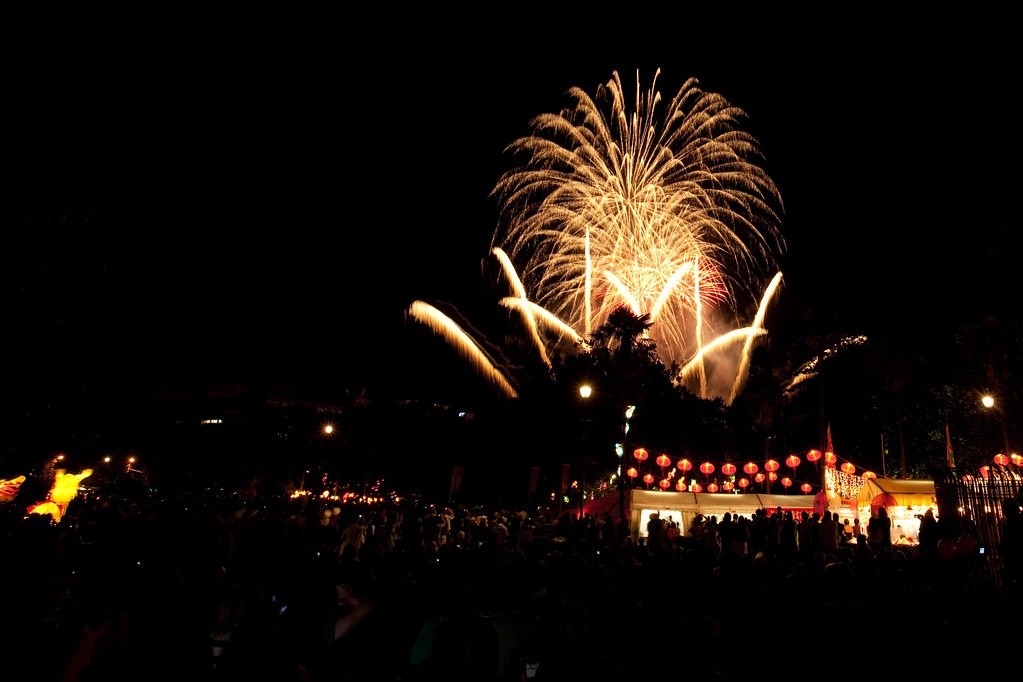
[945,424,959,481]
[562,465,572,493]
[530,466,541,491]
[823,426,835,468]
[450,467,464,491]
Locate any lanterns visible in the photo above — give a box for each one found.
[963,451,1023,483]
[627,449,877,491]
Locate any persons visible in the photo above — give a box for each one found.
[0,485,1023,682]
